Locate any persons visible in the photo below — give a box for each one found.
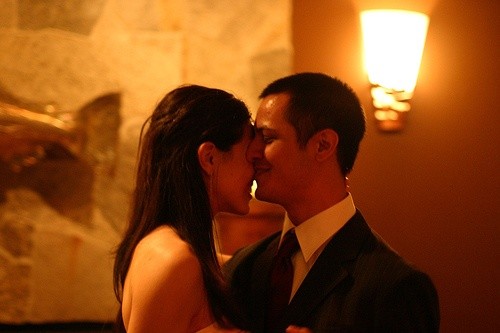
[107,82,350,333]
[216,71,440,333]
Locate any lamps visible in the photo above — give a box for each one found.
[358,8,430,130]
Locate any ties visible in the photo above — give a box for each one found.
[268,228,300,332]
